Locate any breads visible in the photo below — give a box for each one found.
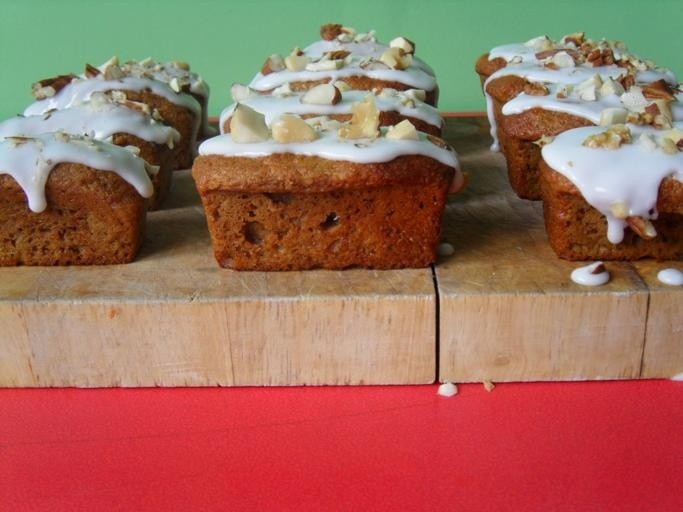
[475,32,681,264]
[191,24,459,272]
[2,54,212,265]
